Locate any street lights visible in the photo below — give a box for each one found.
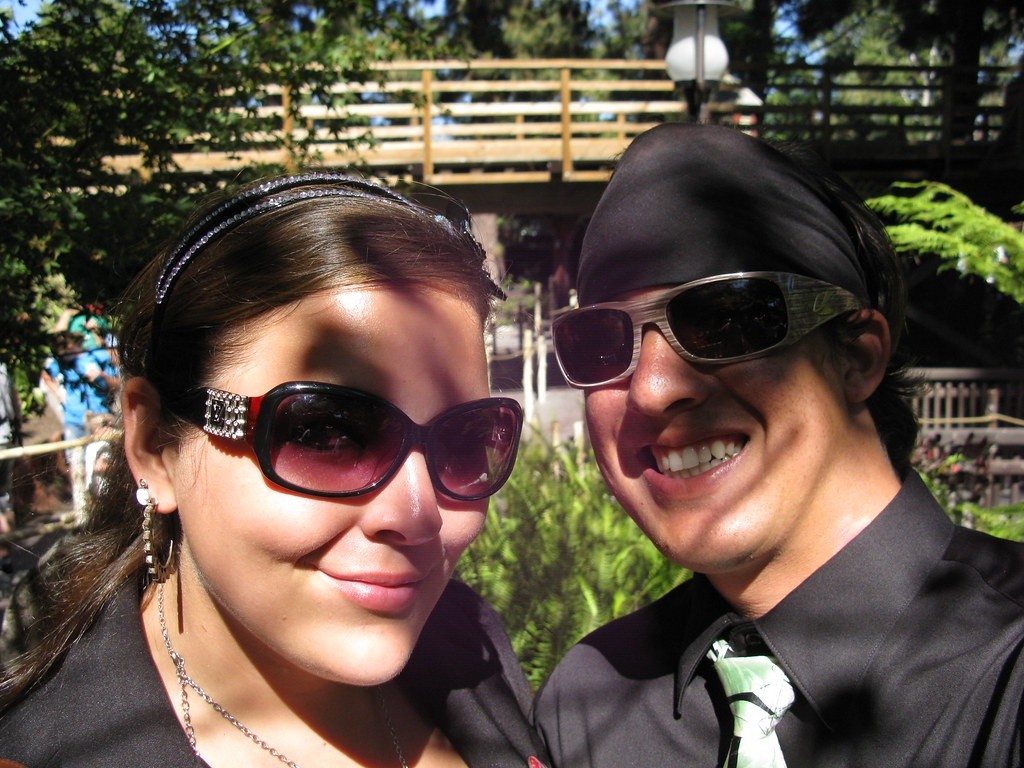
[651,1,740,123]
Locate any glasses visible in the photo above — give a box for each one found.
[551,271,863,388]
[168,380,525,501]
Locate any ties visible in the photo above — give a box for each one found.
[707,638,796,768]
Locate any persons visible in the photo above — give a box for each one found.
[0,291,121,531]
[0,169,552,768]
[526,117,1023,768]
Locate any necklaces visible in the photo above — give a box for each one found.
[157,569,416,768]
